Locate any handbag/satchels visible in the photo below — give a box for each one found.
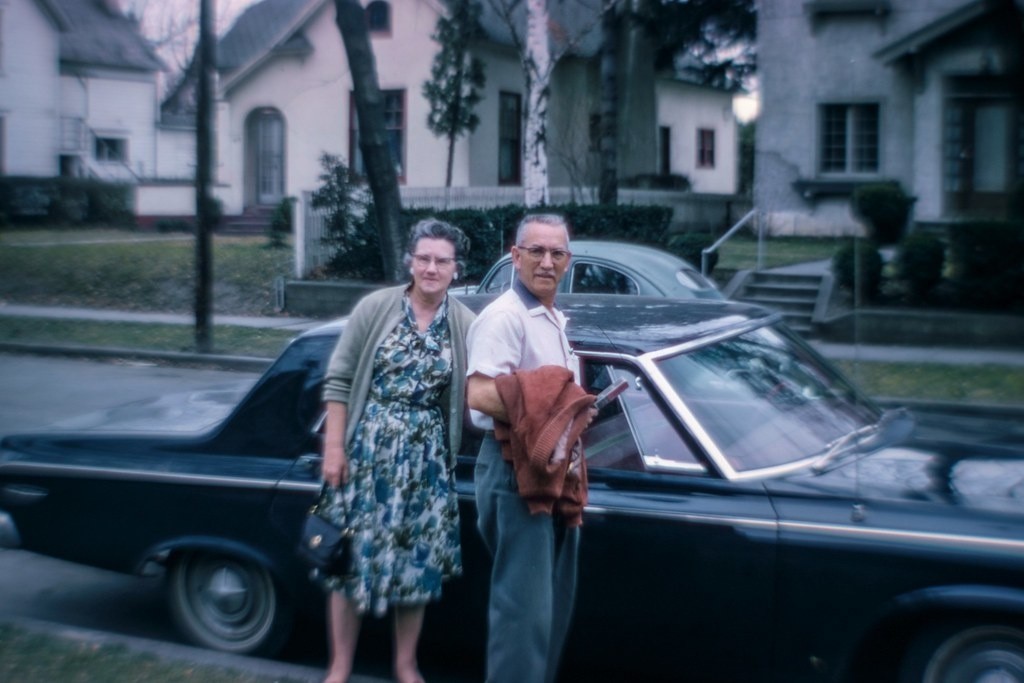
[297,473,356,572]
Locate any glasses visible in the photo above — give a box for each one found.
[518,246,569,262]
[412,253,459,268]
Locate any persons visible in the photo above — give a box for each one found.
[463,211,597,683]
[318,213,482,680]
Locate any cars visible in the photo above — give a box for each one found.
[4,284,1024,683]
[447,243,728,302]
